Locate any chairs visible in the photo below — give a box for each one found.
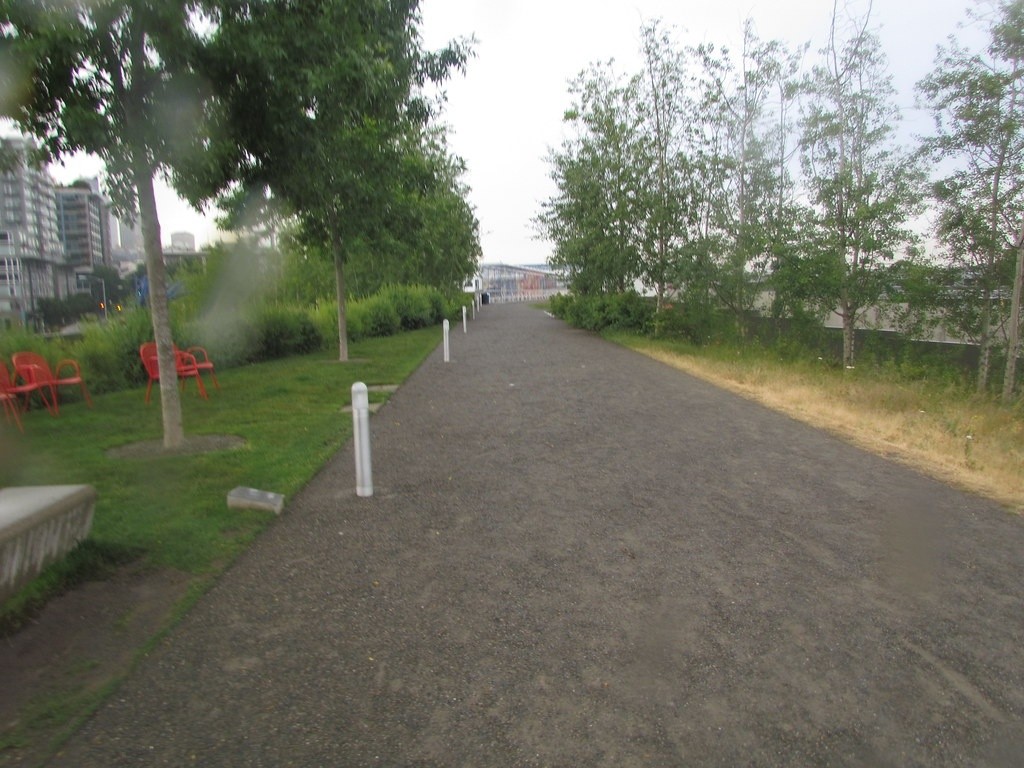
[0,350,95,433]
[140,339,220,401]
